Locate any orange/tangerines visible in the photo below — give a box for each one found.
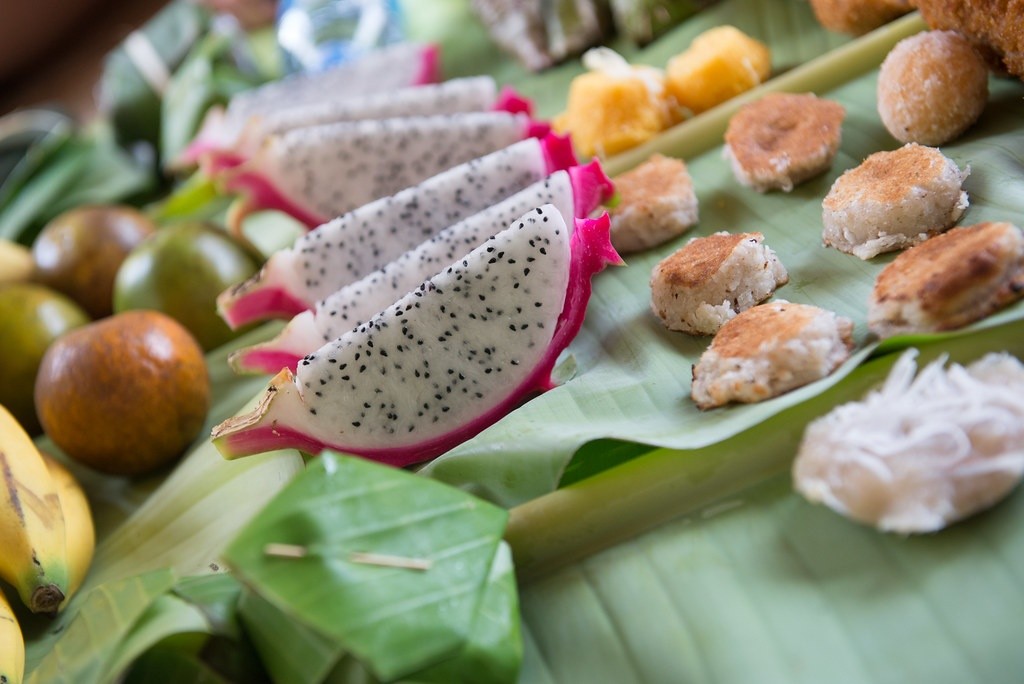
[0,203,254,476]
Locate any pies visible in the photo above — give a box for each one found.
[591,86,1023,409]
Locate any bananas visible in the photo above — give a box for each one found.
[0,402,96,684]
